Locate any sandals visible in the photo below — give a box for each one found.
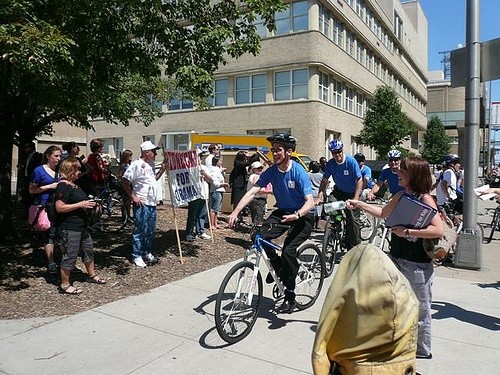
[59,284,83,293]
[89,273,106,284]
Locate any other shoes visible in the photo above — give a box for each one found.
[279,299,297,313]
[71,266,82,273]
[211,224,224,230]
[266,271,274,283]
[48,262,60,273]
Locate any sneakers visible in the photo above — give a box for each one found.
[187,235,195,242]
[143,252,160,263]
[132,255,147,268]
[196,233,211,240]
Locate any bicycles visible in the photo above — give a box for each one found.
[92,171,135,234]
[312,196,395,278]
[486,196,500,244]
[433,186,485,266]
[214,213,327,345]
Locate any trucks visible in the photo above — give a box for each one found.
[189,132,313,175]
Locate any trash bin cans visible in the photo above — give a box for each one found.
[365,160,391,200]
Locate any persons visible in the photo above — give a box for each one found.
[314,139,363,252]
[344,156,445,359]
[54,156,106,294]
[184,142,500,249]
[84,138,112,216]
[121,141,164,267]
[117,150,133,219]
[227,133,314,313]
[60,139,90,194]
[29,145,64,273]
[17,142,41,209]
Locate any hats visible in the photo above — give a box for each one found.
[140,140,160,151]
[251,162,263,170]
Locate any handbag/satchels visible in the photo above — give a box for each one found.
[418,195,457,265]
[28,204,51,231]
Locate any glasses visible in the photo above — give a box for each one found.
[332,151,342,155]
[389,159,398,162]
[270,147,290,153]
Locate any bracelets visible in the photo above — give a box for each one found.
[295,211,300,218]
[158,171,162,174]
[406,228,410,239]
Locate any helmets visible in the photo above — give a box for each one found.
[445,154,461,162]
[354,153,365,160]
[387,150,403,158]
[267,133,297,150]
[328,139,343,152]
